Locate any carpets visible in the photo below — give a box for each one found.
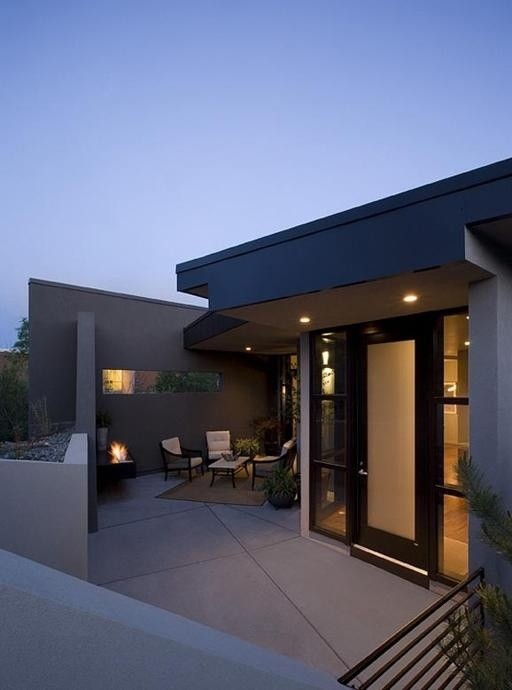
[155,461,274,507]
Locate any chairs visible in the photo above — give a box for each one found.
[202,429,238,472]
[158,436,205,484]
[249,432,297,491]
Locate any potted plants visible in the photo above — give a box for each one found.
[257,466,298,511]
[95,405,110,453]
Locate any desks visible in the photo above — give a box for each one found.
[95,449,136,497]
